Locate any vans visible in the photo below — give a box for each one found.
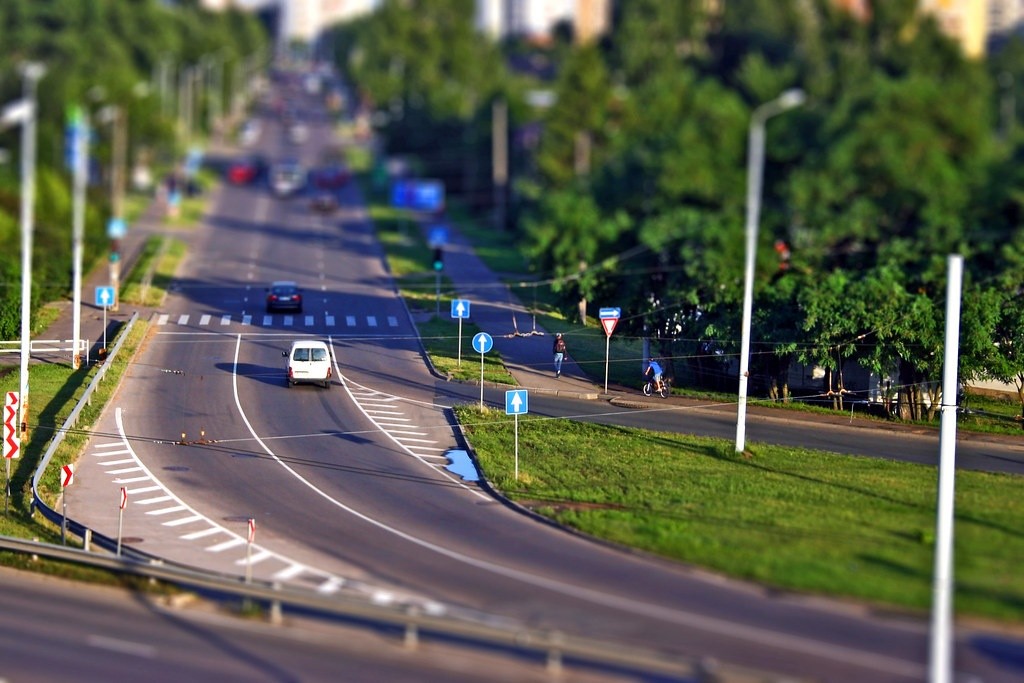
[281,340,332,389]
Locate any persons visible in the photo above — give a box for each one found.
[645,358,662,392]
[553,333,567,378]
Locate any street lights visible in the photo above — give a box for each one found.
[735,88,807,452]
[20,57,60,442]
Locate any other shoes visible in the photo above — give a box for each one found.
[655,389,662,392]
[557,370,560,374]
[557,375,560,378]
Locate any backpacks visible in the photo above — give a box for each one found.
[557,340,566,352]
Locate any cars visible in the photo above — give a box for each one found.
[264,281,304,315]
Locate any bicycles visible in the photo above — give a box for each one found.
[643,375,669,399]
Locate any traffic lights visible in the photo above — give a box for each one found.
[432,248,444,272]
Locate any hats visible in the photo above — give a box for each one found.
[556,333,561,337]
